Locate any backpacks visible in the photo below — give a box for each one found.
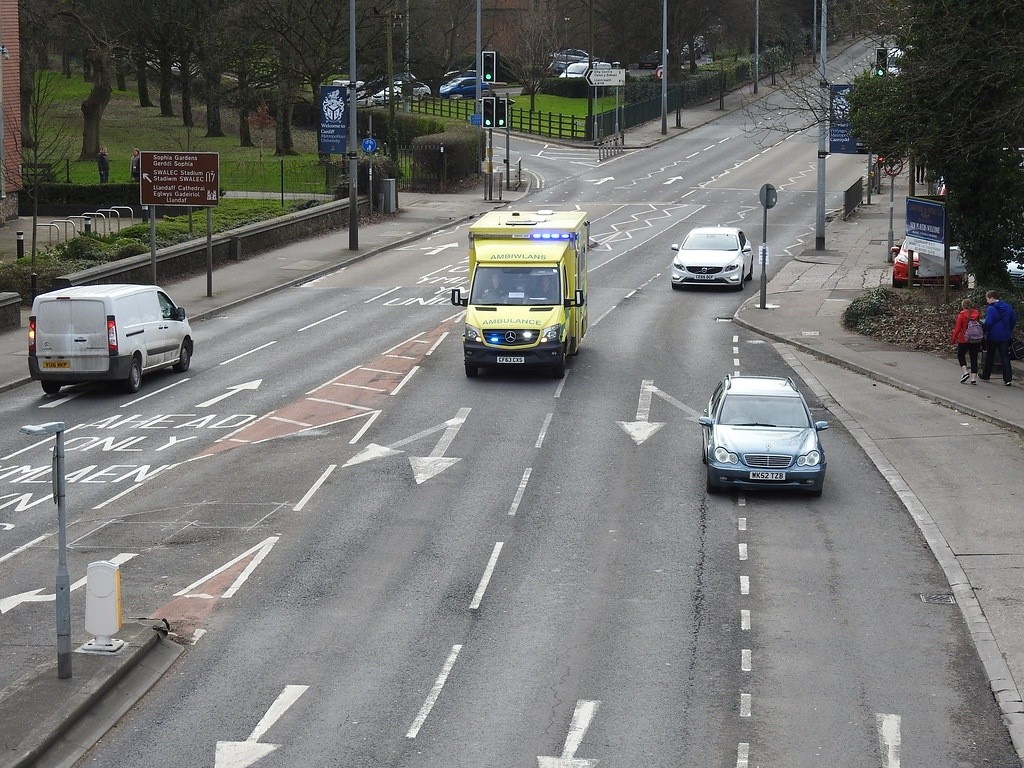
[961,320,983,343]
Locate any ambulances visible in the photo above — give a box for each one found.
[451,209,590,379]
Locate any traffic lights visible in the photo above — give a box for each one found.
[482,51,496,83]
[876,47,888,78]
[494,98,506,128]
[481,97,495,128]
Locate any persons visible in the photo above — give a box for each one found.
[98,147,110,182]
[535,277,555,299]
[132,149,140,180]
[951,300,980,384]
[485,273,505,296]
[916,158,924,185]
[977,291,1016,386]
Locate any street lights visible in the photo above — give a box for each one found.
[564,17,571,79]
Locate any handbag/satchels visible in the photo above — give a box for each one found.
[1009,337,1024,360]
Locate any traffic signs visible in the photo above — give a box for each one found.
[585,67,626,86]
[139,150,219,207]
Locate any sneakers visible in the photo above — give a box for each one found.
[972,380,976,385]
[960,373,970,382]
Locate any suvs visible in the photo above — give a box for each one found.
[698,373,830,496]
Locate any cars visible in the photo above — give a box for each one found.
[869,45,914,78]
[670,224,753,291]
[890,234,1024,290]
[332,68,492,107]
[546,48,601,77]
[638,49,663,70]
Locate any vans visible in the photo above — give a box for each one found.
[558,59,613,80]
[27,283,195,394]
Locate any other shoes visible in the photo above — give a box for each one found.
[1003,381,1011,385]
[978,373,989,382]
